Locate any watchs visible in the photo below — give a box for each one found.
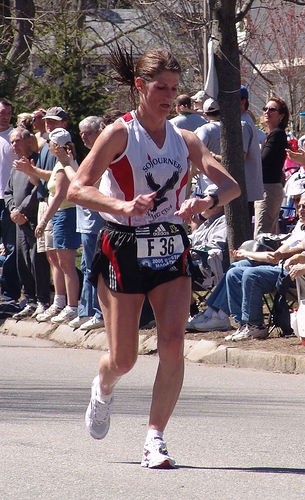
[202,192,219,209]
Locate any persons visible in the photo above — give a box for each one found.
[185,190,305,342]
[241,84,264,223]
[253,96,289,240]
[169,90,221,196]
[276,111,305,234]
[67,49,242,469]
[55,106,128,330]
[186,184,229,316]
[0,98,78,324]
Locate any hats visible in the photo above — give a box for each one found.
[191,91,210,103]
[41,106,70,122]
[240,84,248,101]
[48,127,71,147]
[203,98,220,113]
[287,179,305,196]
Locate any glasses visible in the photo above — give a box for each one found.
[79,127,98,136]
[262,106,280,114]
[297,204,304,208]
[46,139,51,143]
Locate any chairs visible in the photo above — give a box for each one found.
[188,242,229,306]
[258,233,305,337]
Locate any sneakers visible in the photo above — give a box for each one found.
[68,316,91,328]
[185,311,210,330]
[80,317,105,329]
[224,324,246,341]
[35,305,64,321]
[51,306,78,322]
[12,302,36,320]
[84,375,113,440]
[232,323,268,339]
[31,302,50,317]
[142,438,176,468]
[193,311,231,330]
